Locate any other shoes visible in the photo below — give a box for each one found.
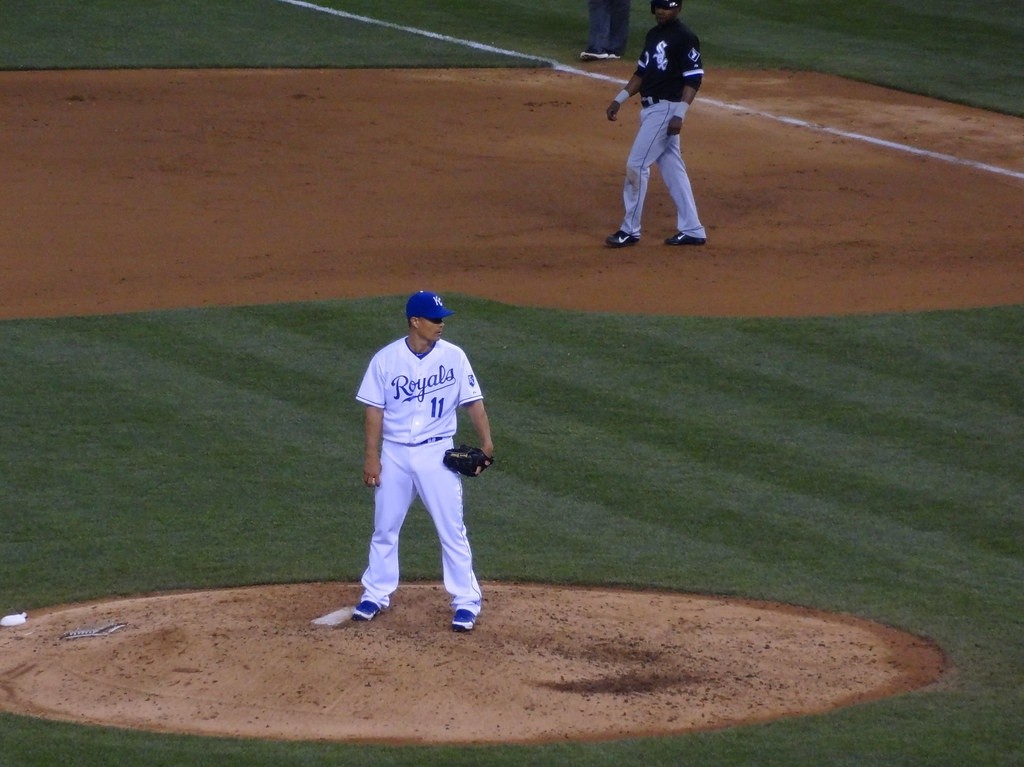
[606,231,639,247]
[608,54,621,60]
[664,232,706,245]
[580,48,609,61]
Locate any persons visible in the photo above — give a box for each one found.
[579,0,631,60]
[352,291,493,631]
[606,0,706,248]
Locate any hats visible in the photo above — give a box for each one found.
[652,0,682,12]
[406,291,457,319]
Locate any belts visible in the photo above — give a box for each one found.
[641,98,659,108]
[421,437,442,444]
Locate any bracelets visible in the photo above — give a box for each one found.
[613,90,630,105]
[673,101,690,122]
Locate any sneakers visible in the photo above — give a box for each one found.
[353,600,381,621]
[452,609,476,630]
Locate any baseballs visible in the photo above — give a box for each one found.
[371,477,376,486]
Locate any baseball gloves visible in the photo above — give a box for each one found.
[442,444,495,478]
